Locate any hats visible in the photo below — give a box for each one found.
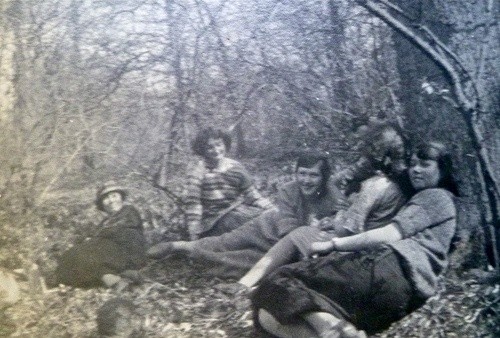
[96,182,126,212]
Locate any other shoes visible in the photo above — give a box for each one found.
[323,319,366,338]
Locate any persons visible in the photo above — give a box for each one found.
[147,127,457,338]
[54,178,150,296]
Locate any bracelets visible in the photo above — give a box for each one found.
[332,239,341,250]
